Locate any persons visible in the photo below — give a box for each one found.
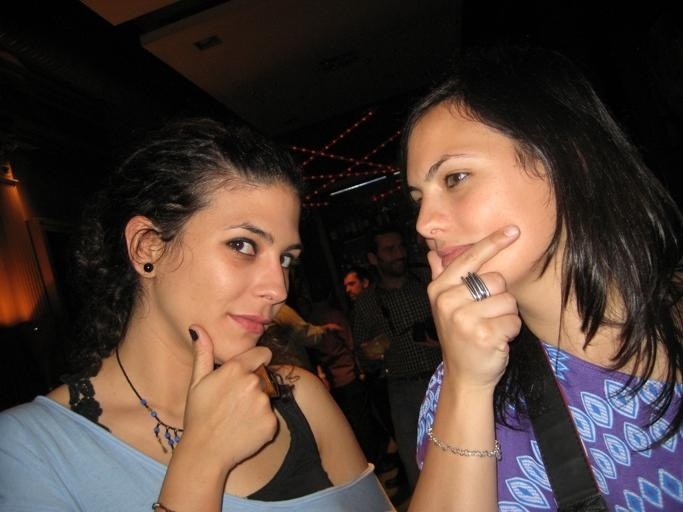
[260,227,436,490]
[0,112,397,511]
[405,48,683,512]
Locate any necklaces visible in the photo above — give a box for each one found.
[115,346,184,454]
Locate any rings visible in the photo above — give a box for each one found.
[460,270,490,301]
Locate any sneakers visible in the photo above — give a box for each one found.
[384,465,411,506]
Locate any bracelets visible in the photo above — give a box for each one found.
[428,426,501,460]
